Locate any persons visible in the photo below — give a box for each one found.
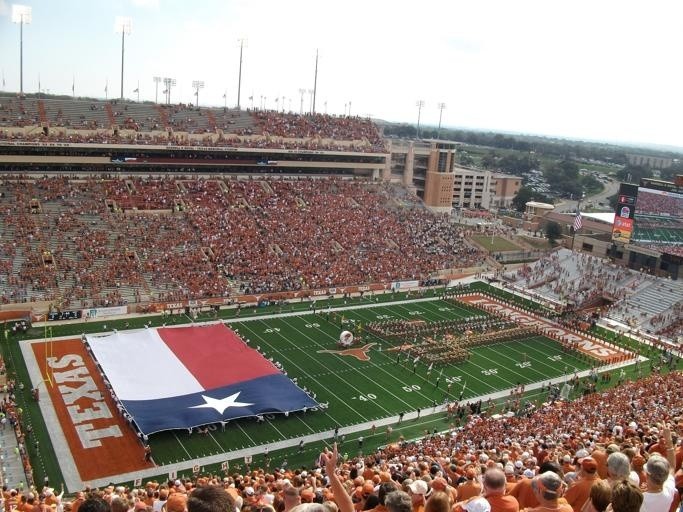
[1,96,682,512]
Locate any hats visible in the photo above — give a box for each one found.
[150,441,670,494]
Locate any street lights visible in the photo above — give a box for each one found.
[11,3,33,94]
[113,14,132,99]
